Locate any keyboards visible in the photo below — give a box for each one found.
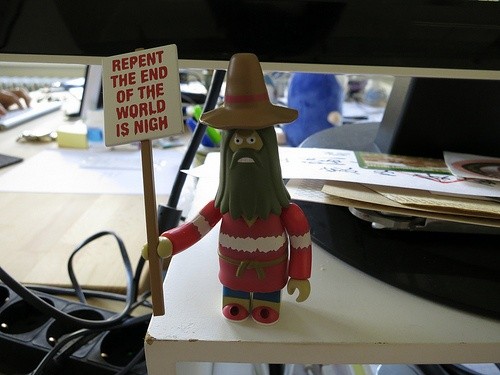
[0,101,60,130]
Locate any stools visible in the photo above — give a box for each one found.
[377,363,500,375]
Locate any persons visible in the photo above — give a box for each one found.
[0,88,32,117]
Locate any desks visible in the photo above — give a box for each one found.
[0,76,226,315]
[144,152,500,375]
